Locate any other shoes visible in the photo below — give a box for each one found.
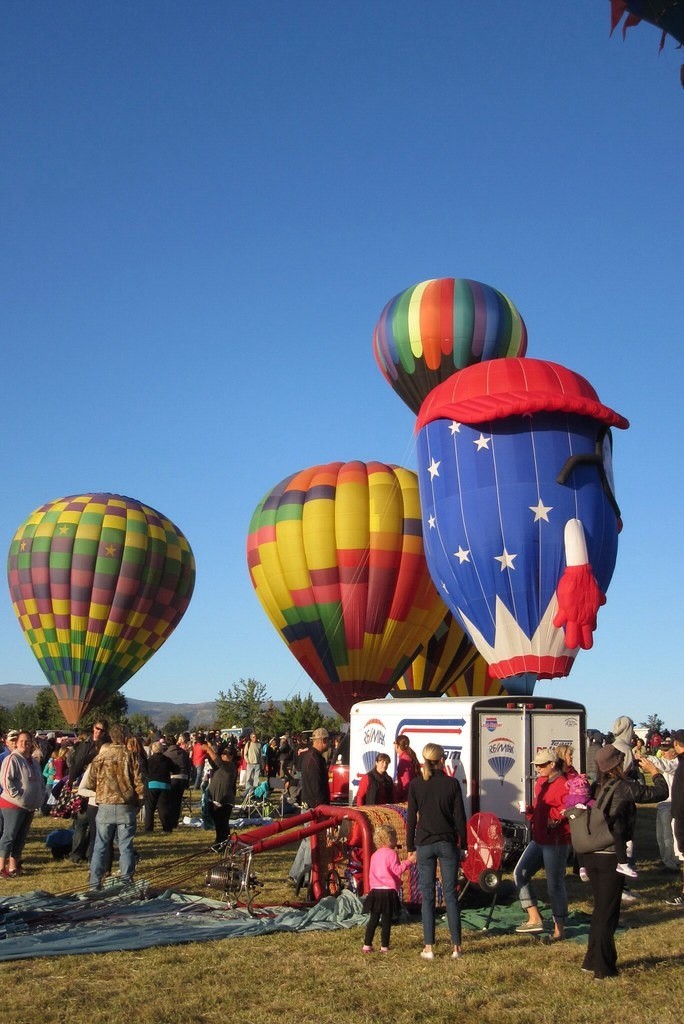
[621,891,636,900]
[287,877,296,886]
[594,973,621,981]
[581,965,594,974]
[0,869,13,879]
[660,867,680,874]
[541,935,563,942]
[13,867,23,878]
[420,949,434,959]
[651,862,665,868]
[451,952,460,958]
[579,867,590,882]
[380,946,388,952]
[515,923,544,933]
[361,945,373,953]
[616,863,638,878]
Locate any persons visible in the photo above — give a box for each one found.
[405,740,467,960]
[584,713,684,905]
[0,727,86,878]
[360,825,417,954]
[286,727,346,889]
[355,753,394,806]
[392,735,423,803]
[68,717,192,893]
[179,726,296,852]
[511,744,670,983]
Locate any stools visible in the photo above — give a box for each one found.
[181,789,192,816]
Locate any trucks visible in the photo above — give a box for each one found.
[327,695,587,867]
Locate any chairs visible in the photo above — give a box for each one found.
[441,811,544,931]
[248,778,288,819]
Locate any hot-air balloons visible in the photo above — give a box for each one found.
[247,460,450,724]
[7,494,196,739]
[373,278,527,418]
[389,610,508,697]
[413,358,630,694]
[0,802,446,962]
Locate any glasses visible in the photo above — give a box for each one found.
[94,725,106,733]
[392,741,399,745]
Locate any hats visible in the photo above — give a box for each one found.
[593,744,625,773]
[4,729,19,741]
[279,735,287,739]
[565,774,592,795]
[659,740,674,751]
[530,749,560,765]
[309,728,330,739]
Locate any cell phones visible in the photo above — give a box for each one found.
[634,759,642,766]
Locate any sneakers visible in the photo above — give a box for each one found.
[666,893,684,905]
